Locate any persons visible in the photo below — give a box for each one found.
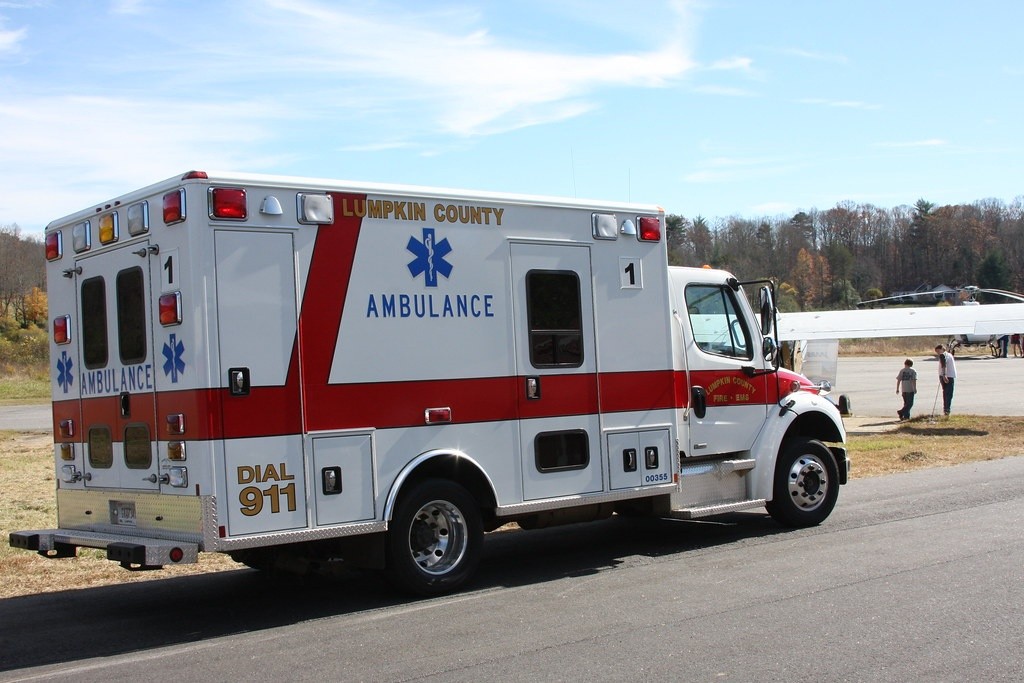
[935,344,956,416]
[989,334,1024,358]
[946,336,958,355]
[896,358,917,421]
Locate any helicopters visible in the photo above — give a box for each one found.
[856,286,1024,357]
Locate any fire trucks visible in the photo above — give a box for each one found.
[9,171,852,595]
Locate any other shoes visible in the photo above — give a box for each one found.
[898,410,904,420]
